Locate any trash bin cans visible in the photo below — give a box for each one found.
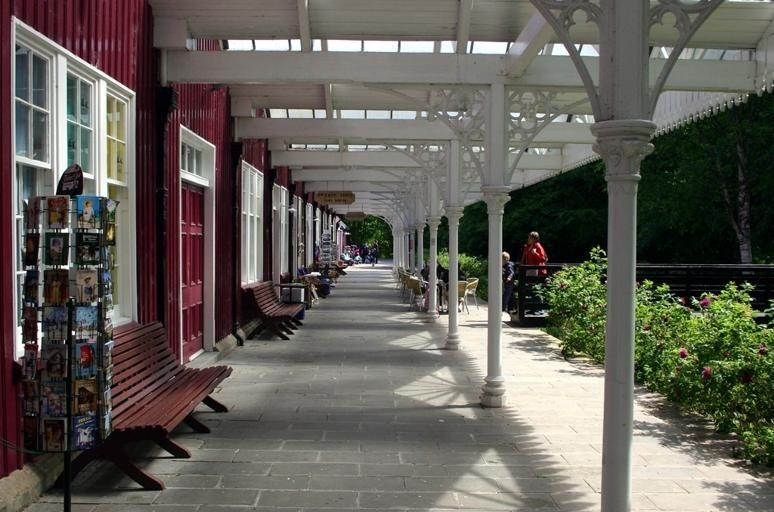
[278,283,312,307]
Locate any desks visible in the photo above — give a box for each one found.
[275,283,306,304]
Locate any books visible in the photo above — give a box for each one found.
[18,193,119,453]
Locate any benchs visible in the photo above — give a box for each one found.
[242,279,307,341]
[46,316,232,492]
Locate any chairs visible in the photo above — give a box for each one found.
[397,266,480,316]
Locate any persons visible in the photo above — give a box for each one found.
[345,237,380,265]
[418,258,466,314]
[502,250,519,314]
[519,231,547,315]
